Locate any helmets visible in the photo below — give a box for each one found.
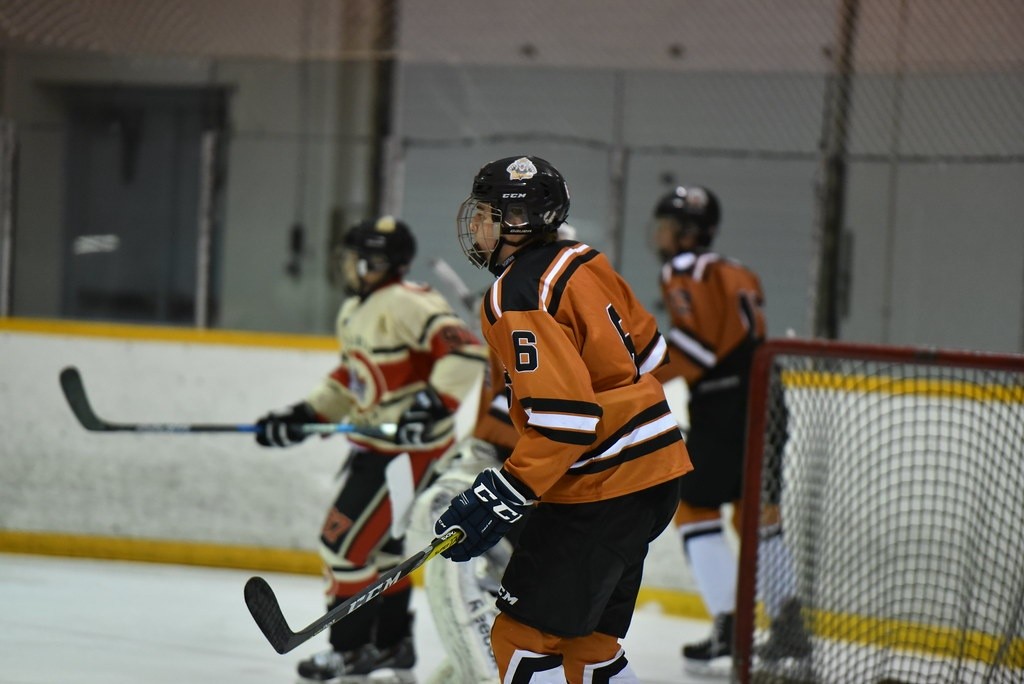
[654,186,720,247]
[344,217,416,286]
[458,156,570,271]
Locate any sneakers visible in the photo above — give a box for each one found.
[751,596,813,680]
[683,610,739,676]
[297,643,372,684]
[327,634,414,684]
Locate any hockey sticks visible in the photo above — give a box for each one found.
[432,254,490,316]
[238,530,463,658]
[56,364,398,442]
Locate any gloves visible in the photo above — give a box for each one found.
[254,403,318,448]
[432,469,535,561]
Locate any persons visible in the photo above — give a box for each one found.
[647,183,813,670]
[258,216,486,684]
[410,155,694,684]
